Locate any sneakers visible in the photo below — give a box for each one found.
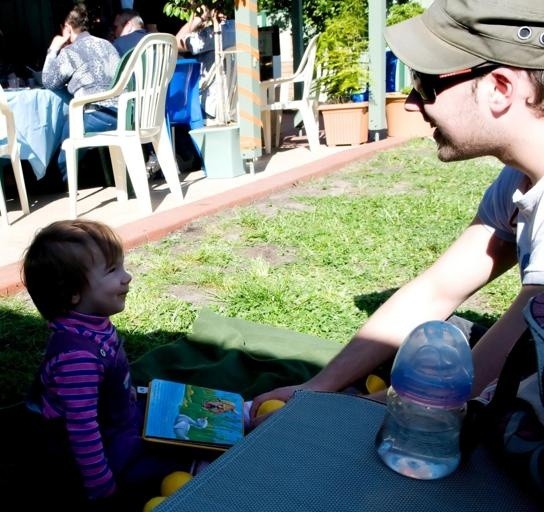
[146,151,160,179]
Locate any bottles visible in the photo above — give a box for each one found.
[374,320,477,480]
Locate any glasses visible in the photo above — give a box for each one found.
[411,62,499,103]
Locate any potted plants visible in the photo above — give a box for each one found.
[387,3,434,138]
[313,1,369,149]
[164,1,246,178]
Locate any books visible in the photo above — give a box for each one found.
[143,378,245,451]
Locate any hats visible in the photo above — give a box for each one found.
[384,0,544,75]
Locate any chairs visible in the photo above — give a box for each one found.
[201,51,238,121]
[59,33,183,218]
[260,32,322,153]
[0,91,32,223]
[163,58,206,165]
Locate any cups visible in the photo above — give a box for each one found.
[27,78,33,89]
[7,77,19,89]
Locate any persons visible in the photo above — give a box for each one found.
[40,0,235,190]
[22,220,193,511]
[250,1,544,495]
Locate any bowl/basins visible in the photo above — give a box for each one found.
[33,70,42,86]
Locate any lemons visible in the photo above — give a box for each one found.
[366,374,387,394]
[256,399,286,418]
[144,496,167,512]
[160,471,194,496]
